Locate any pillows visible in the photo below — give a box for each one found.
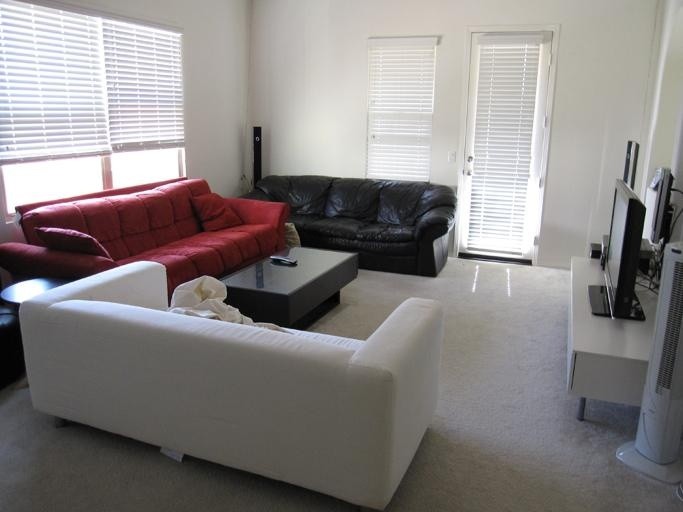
[189,193,243,230]
[33,227,110,257]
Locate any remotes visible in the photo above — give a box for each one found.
[269,253,297,267]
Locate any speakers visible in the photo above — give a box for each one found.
[253,126,262,195]
[624,141,639,190]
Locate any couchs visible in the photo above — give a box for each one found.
[238,175,457,278]
[19,261,442,511]
[0,177,290,296]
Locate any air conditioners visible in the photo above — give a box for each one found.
[615,242,683,485]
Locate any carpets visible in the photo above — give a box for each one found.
[0,256,683,512]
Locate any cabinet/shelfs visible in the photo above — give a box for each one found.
[567,255,659,422]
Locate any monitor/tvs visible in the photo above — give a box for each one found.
[647,169,674,243]
[587,179,648,322]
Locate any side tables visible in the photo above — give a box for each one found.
[0,278,72,306]
